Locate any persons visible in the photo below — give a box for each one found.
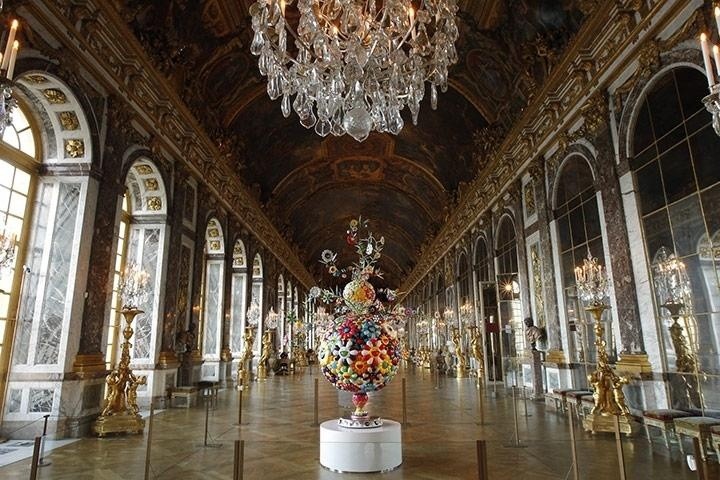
[611,375,631,416]
[682,371,707,409]
[238,336,253,370]
[587,370,603,415]
[452,334,465,365]
[184,323,196,347]
[470,331,482,361]
[524,318,544,344]
[101,369,125,417]
[258,334,271,368]
[127,370,146,417]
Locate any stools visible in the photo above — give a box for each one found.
[552,388,594,419]
[641,408,720,466]
[170,379,220,409]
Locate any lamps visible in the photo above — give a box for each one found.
[572,246,634,437]
[413,298,485,383]
[650,244,708,408]
[248,0,463,142]
[235,296,311,390]
[94,261,148,436]
